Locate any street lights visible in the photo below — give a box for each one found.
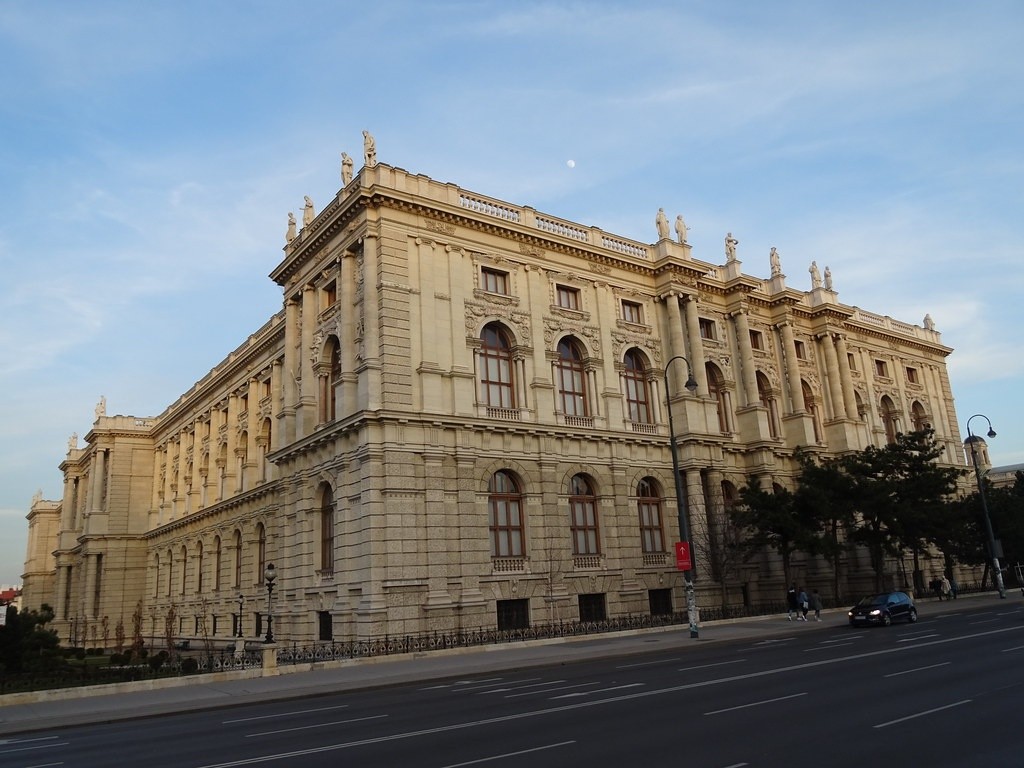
[967,413,1007,600]
[664,356,703,638]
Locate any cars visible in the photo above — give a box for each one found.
[848,590,919,629]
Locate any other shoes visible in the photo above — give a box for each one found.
[788,616,791,621]
[814,615,816,620]
[818,619,822,622]
[797,617,802,621]
[802,616,806,620]
[805,619,809,622]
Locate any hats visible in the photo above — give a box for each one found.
[933,575,938,577]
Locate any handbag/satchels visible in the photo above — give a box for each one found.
[950,591,954,597]
[803,601,809,609]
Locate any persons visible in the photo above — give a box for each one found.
[932,575,957,601]
[787,586,822,622]
